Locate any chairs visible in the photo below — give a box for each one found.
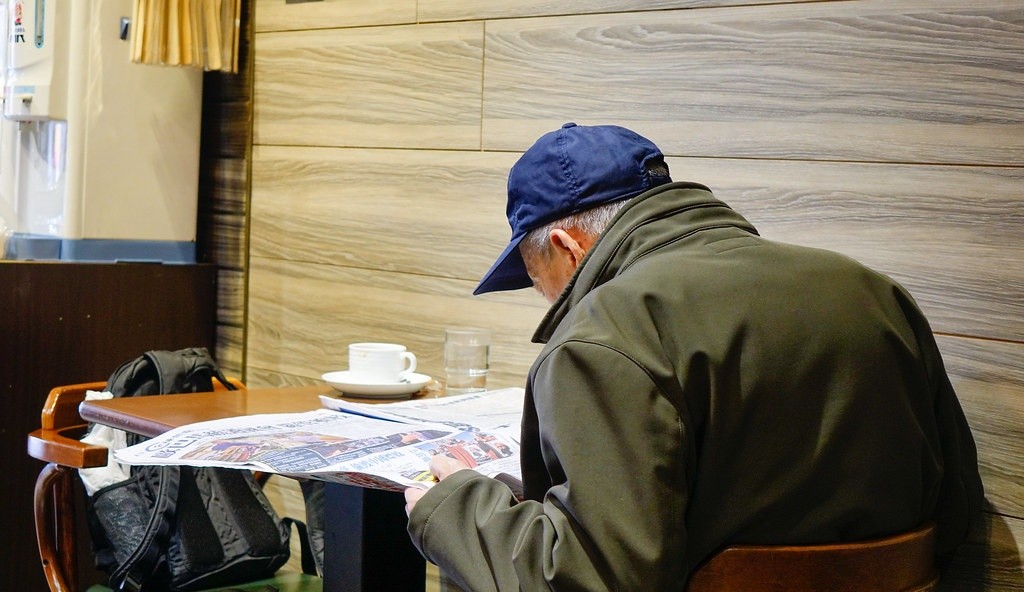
[26,377,323,592]
[686,518,943,592]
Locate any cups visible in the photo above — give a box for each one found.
[348,342,417,383]
[444,328,489,396]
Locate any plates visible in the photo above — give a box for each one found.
[322,371,431,397]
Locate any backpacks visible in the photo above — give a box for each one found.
[77,346,291,592]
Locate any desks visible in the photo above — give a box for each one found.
[79,385,426,592]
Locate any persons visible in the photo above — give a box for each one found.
[388,425,450,448]
[428,432,513,462]
[403,120,985,592]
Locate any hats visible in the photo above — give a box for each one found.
[472,122,669,296]
